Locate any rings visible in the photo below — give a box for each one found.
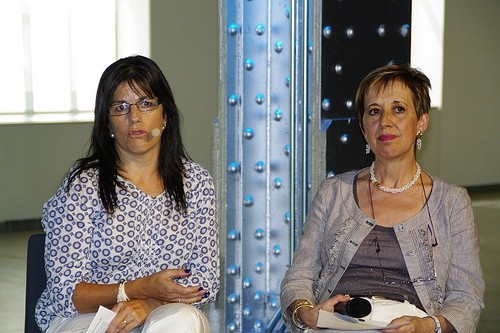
[178,299,180,303]
[123,319,128,325]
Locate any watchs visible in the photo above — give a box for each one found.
[430,315,442,333]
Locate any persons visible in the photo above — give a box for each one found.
[280,65,485,333]
[35,56,220,333]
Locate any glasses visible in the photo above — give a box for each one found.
[109,96,162,116]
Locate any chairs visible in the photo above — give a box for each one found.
[25,234,47,333]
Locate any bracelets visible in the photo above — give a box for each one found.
[294,301,310,324]
[292,305,314,329]
[116,281,130,302]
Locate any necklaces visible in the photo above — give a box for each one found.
[369,161,421,193]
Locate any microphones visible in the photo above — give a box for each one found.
[334,298,372,318]
